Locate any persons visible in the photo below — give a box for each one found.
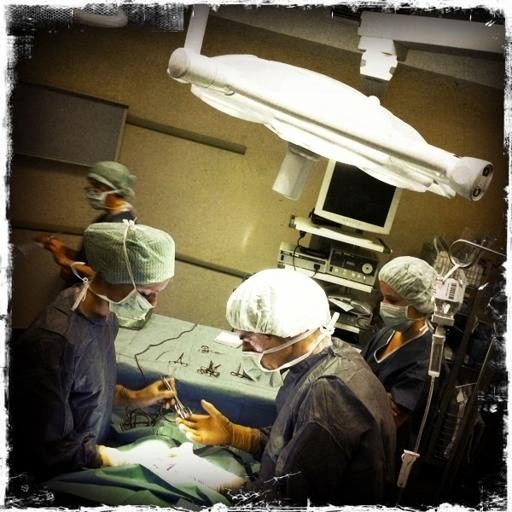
[173,267,399,507]
[360,256,456,505]
[33,160,140,289]
[11,221,177,502]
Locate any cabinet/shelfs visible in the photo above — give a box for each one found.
[111,303,292,425]
[275,214,391,337]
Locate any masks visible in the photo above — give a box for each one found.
[239,351,284,388]
[378,301,416,334]
[86,192,106,211]
[107,291,156,328]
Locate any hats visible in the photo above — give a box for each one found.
[226,269,332,339]
[87,161,137,200]
[83,221,175,285]
[379,256,437,314]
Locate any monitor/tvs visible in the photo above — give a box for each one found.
[312,157,404,243]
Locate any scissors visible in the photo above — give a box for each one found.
[230,363,255,383]
[169,352,188,367]
[197,361,222,377]
[197,345,223,355]
[160,375,192,420]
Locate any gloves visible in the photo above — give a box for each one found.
[176,399,261,456]
[113,377,175,410]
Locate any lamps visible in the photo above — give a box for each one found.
[164,3,494,202]
